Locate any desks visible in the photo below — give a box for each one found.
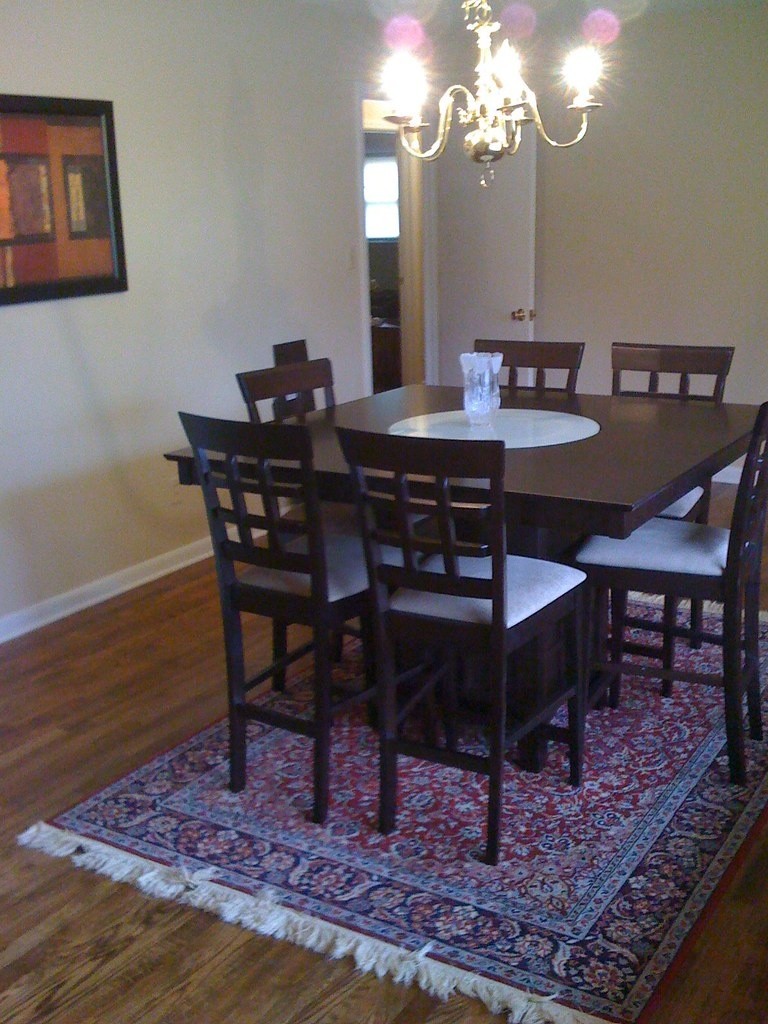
[164,384,759,768]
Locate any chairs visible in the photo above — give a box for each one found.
[177,340,768,865]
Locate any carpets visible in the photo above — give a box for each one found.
[16,588,768,1024]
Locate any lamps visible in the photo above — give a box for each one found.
[383,0,603,187]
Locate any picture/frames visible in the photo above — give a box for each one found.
[0,94,128,304]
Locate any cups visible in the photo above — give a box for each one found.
[460,352,503,426]
